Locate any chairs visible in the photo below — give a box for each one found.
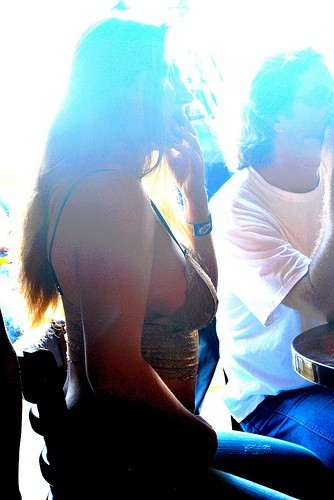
[14,317,187,500]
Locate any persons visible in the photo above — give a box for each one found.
[18,19,334,500]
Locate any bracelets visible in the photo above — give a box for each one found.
[183,211,212,236]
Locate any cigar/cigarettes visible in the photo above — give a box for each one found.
[187,115,205,121]
